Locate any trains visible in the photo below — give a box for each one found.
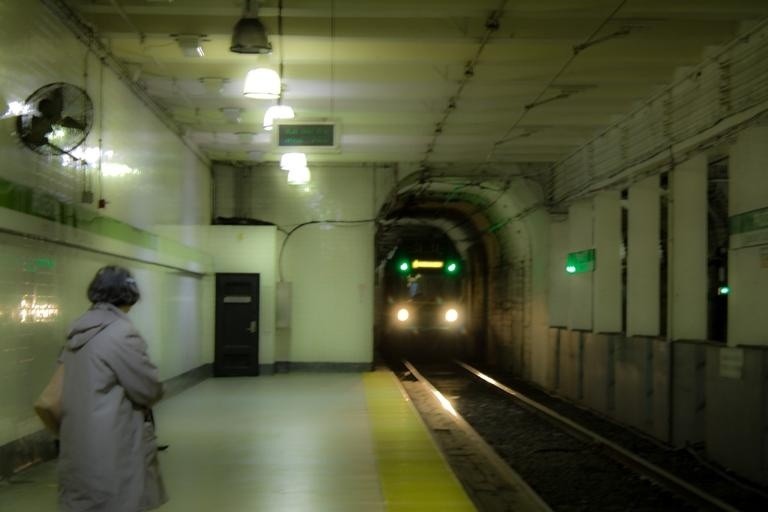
[376,246,471,363]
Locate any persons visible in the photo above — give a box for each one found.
[55,265,170,511]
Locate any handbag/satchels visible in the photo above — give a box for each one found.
[33,362,66,437]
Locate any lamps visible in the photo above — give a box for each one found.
[229,0,273,55]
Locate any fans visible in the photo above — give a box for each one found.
[15,81,95,156]
